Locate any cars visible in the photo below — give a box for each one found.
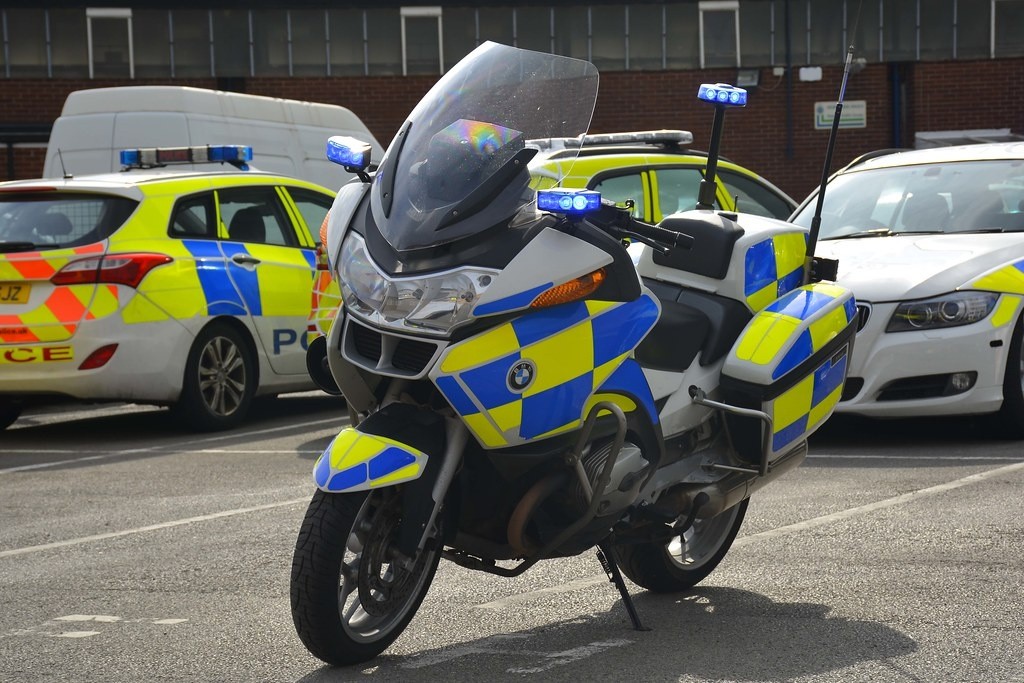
[0,145,335,431]
[528,129,798,222]
[786,140,1023,441]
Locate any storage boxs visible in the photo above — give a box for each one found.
[719,280,859,464]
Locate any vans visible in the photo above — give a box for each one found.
[38,86,386,192]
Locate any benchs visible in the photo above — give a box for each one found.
[951,192,1024,231]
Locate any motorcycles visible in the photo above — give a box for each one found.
[288,44,860,669]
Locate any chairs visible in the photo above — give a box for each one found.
[226,209,266,244]
[900,193,955,230]
[23,212,73,245]
